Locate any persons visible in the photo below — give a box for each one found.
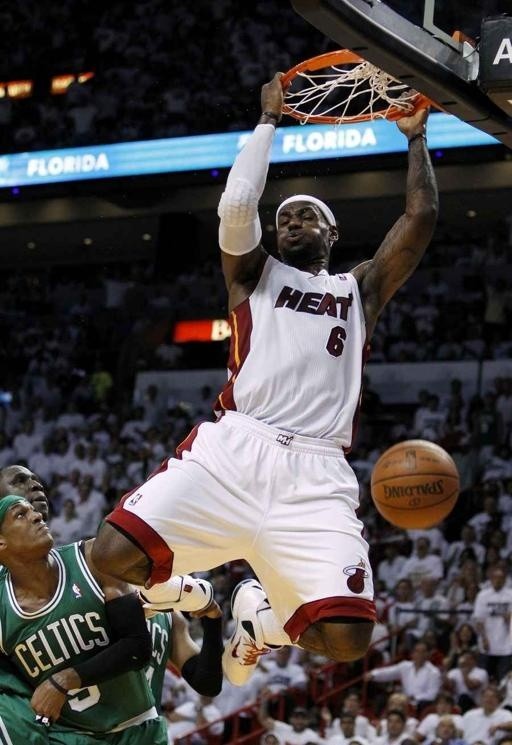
[0,0,512,157]
[0,243,512,744]
[91,68,437,666]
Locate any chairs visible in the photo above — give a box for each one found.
[320,651,462,737]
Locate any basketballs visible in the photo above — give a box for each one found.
[371,439,460,529]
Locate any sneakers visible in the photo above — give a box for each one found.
[132,570,216,614]
[218,575,287,690]
